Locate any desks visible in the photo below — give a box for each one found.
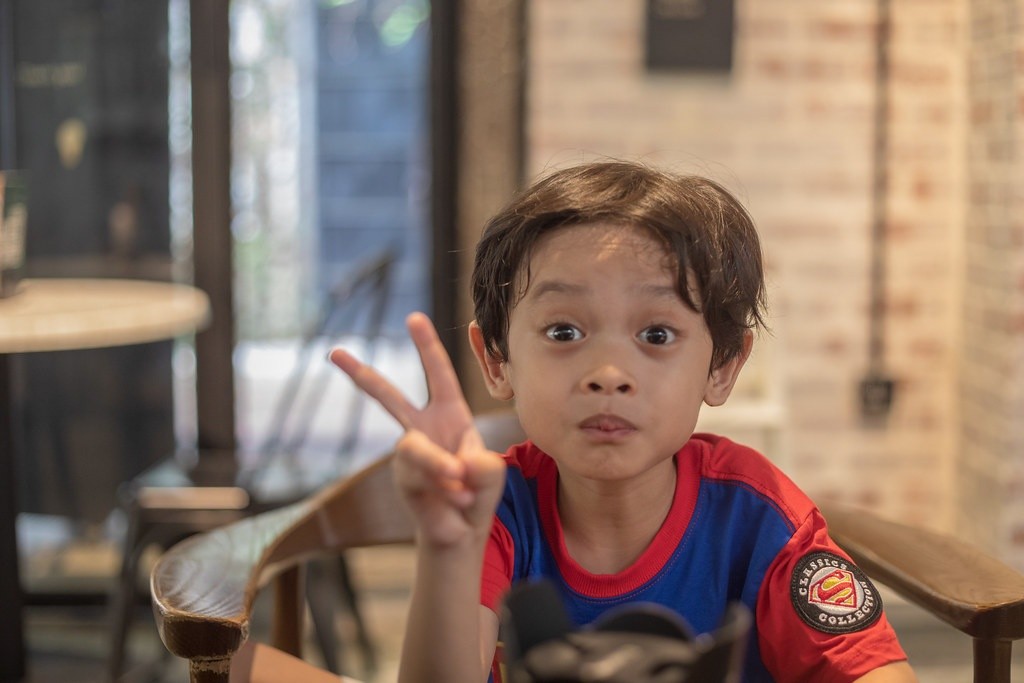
[1,278,211,683]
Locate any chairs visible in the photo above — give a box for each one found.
[149,407,1024,683]
[106,252,399,683]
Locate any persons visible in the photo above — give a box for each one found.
[329,156,920,682]
